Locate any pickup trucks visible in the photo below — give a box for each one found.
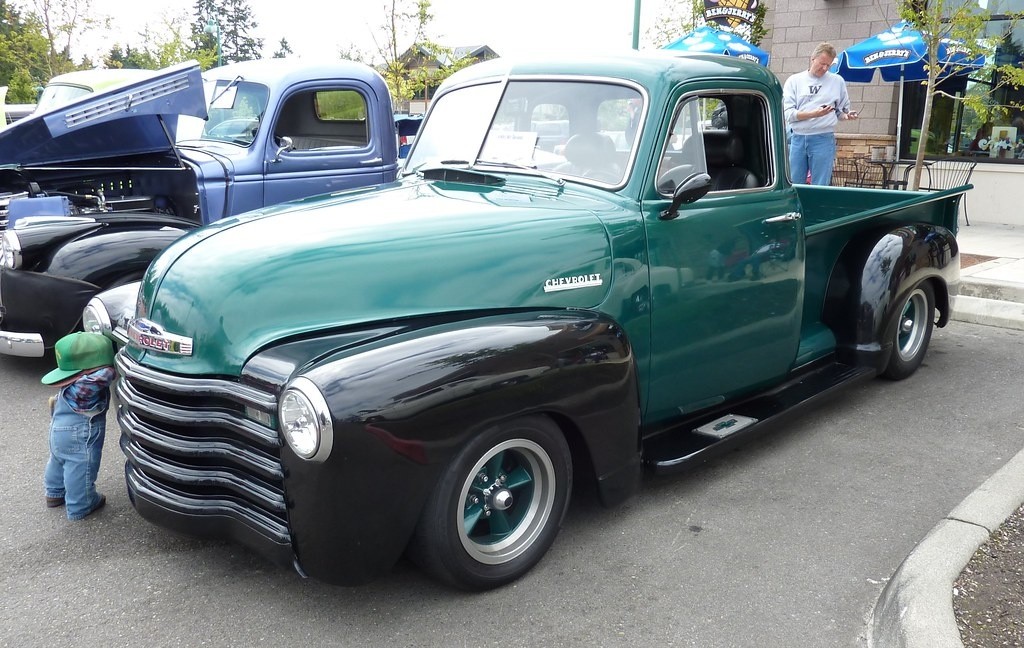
[0,57,574,365]
[112,47,975,596]
[24,67,239,151]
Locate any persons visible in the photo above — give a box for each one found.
[1012,113,1024,143]
[42,332,117,520]
[783,43,858,186]
[964,122,995,156]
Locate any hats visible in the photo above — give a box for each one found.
[41,331,115,388]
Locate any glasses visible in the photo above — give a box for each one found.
[842,97,865,118]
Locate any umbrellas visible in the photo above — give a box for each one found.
[660,24,771,131]
[830,15,985,180]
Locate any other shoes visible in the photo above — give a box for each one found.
[46,496,64,508]
[89,495,106,515]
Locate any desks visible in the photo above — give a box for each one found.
[864,160,932,190]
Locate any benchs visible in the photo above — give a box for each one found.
[280,136,364,151]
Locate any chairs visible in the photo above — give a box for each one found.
[901,156,977,226]
[831,155,898,190]
[554,133,623,185]
[672,130,758,192]
